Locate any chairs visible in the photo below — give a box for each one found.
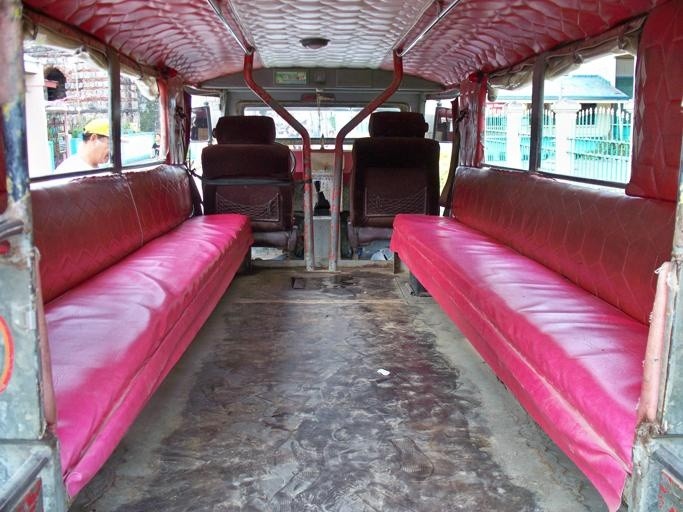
[202,116,301,256]
[346,110,444,254]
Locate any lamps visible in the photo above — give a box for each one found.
[299,36,332,51]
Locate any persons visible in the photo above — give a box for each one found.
[53,118,110,176]
[152,133,161,158]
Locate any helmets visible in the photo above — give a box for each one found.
[82,117,109,137]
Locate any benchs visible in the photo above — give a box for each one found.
[378,162,681,512]
[24,160,252,509]
